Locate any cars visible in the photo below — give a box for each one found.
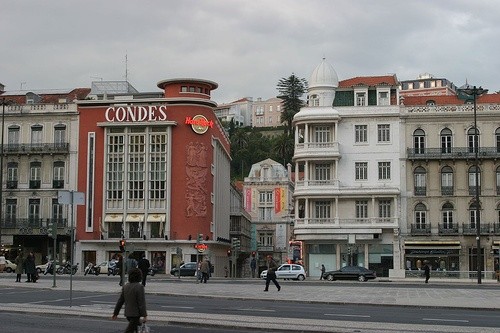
[260,263,307,281]
[322,265,376,282]
[35,259,67,274]
[3,258,17,273]
[170,262,200,276]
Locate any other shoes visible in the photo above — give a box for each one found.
[264,288,268,292]
[277,286,280,291]
[15,278,36,283]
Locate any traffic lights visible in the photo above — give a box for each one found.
[235,239,241,250]
[47,222,56,240]
[231,237,238,249]
[197,232,204,245]
[252,250,255,257]
[227,249,231,257]
[119,239,126,251]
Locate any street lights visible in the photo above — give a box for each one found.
[456,85,489,284]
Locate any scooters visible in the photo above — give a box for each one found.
[106,257,159,276]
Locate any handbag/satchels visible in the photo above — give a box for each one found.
[138,325,150,333]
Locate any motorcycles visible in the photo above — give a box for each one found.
[84,261,101,276]
[44,255,55,275]
[57,258,79,275]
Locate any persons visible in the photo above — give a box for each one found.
[264,255,281,291]
[198,257,212,284]
[251,258,256,278]
[184,141,208,216]
[24,251,36,282]
[424,262,431,283]
[112,268,146,333]
[319,264,326,281]
[124,254,137,268]
[139,254,150,287]
[15,251,24,282]
[115,255,122,286]
[0,254,6,273]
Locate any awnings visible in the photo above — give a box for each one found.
[104,214,124,222]
[146,214,166,222]
[492,246,500,249]
[126,214,145,222]
[402,246,462,249]
